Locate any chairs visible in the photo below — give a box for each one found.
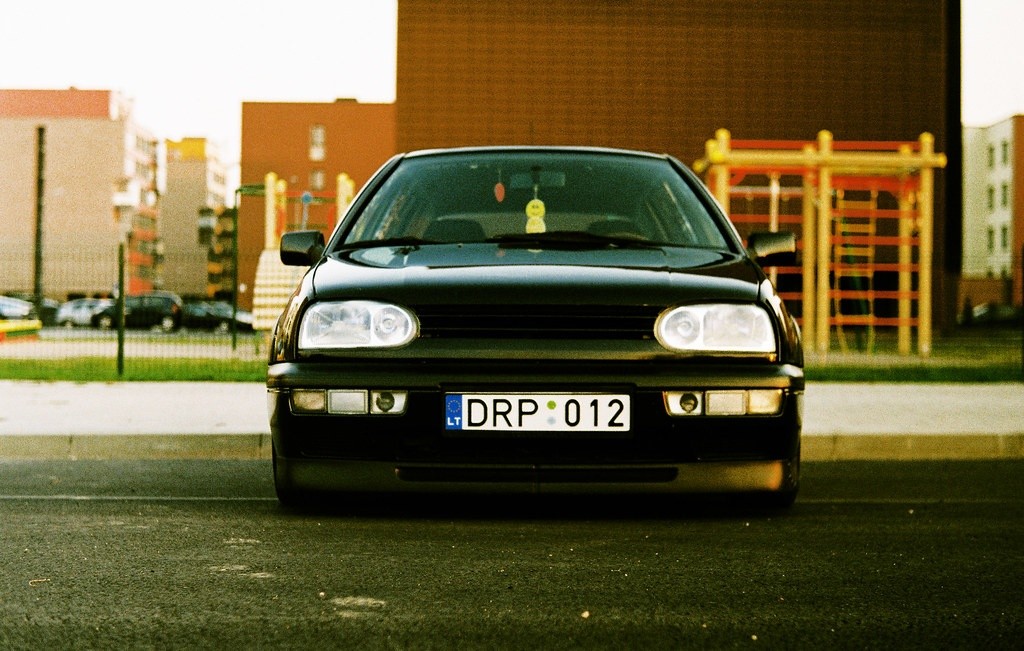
[420,218,484,245]
[586,219,641,238]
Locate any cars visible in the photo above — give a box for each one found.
[181,299,254,334]
[266,145,807,520]
[0,293,117,329]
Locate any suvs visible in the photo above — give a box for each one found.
[91,291,184,331]
